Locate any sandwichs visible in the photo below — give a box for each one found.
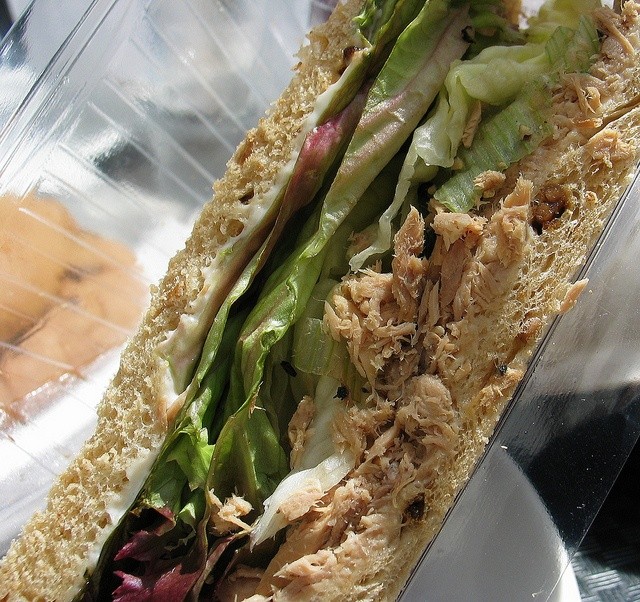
[2,0,638,602]
